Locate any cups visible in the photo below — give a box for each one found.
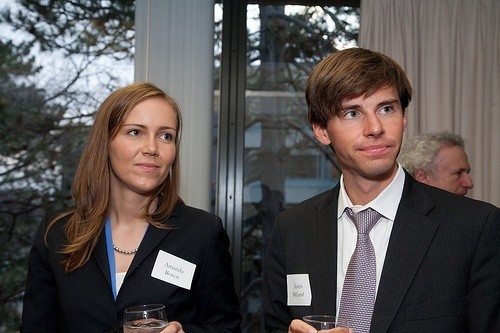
[123,303,168,333]
[302,315,350,333]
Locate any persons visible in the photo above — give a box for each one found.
[400,131,474,198]
[19,82,243,333]
[258,46,499,333]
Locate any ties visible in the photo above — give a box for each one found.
[335,206,384,332]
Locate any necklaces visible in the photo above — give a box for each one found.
[113,244,139,255]
